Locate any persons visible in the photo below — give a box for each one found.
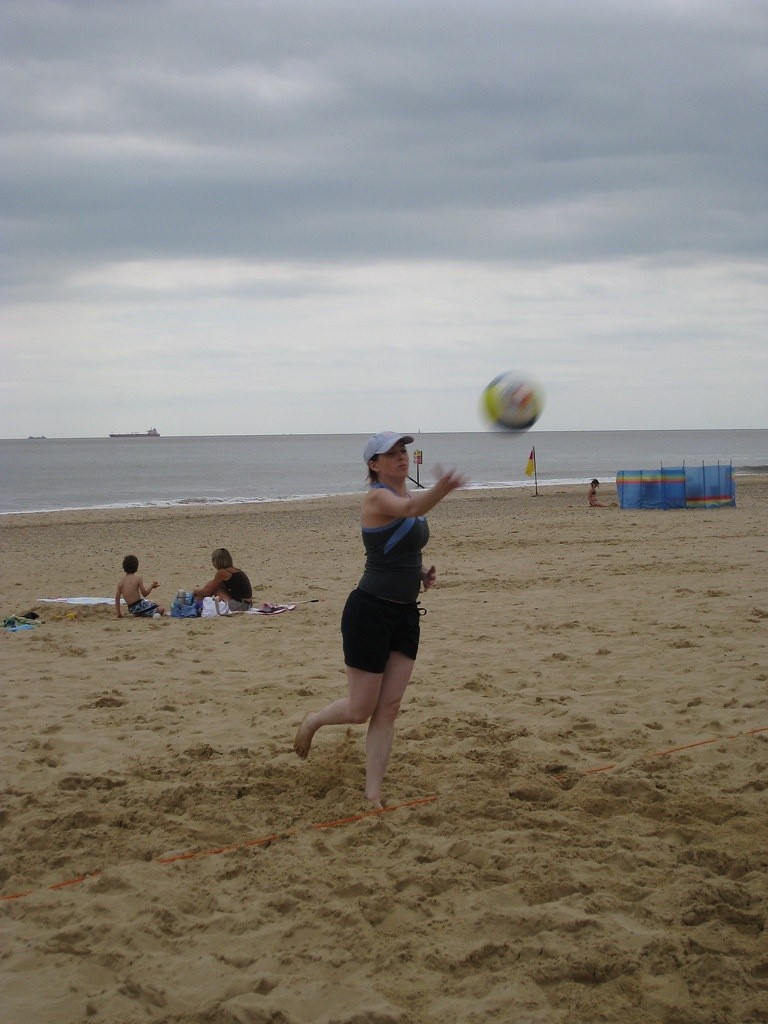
[116,555,164,618]
[192,548,252,611]
[588,479,606,507]
[293,431,462,812]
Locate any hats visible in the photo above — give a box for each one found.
[362,431,414,463]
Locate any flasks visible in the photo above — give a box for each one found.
[177,589,186,605]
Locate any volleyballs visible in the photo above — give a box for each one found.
[477,367,544,437]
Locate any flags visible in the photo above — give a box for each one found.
[525,450,534,477]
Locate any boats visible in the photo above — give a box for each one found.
[109,428,160,437]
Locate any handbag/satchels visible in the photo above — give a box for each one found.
[171,593,204,618]
